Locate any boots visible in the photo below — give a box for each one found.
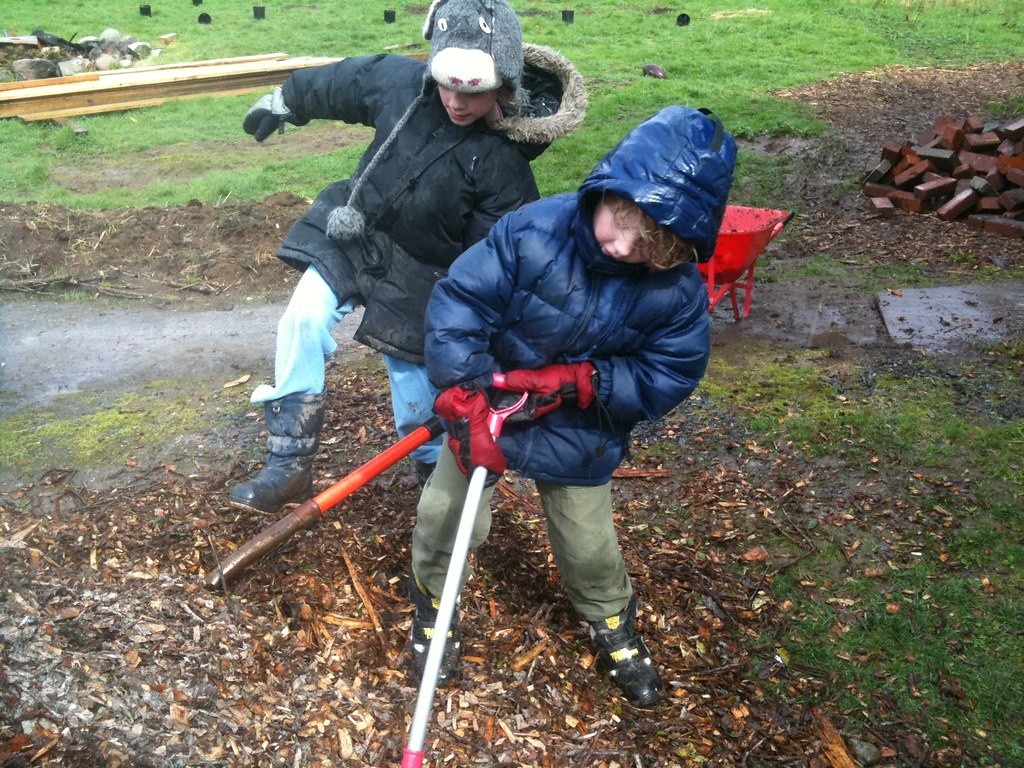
[409,564,462,684]
[588,600,667,708]
[416,461,438,504]
[229,392,324,516]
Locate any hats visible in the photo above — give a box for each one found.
[418,0,524,94]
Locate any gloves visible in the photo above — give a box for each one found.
[485,361,599,426]
[240,90,292,145]
[426,376,510,493]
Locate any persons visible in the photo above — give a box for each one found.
[227,0,587,521]
[409,105,739,706]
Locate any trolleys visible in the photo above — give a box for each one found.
[692,204,795,322]
